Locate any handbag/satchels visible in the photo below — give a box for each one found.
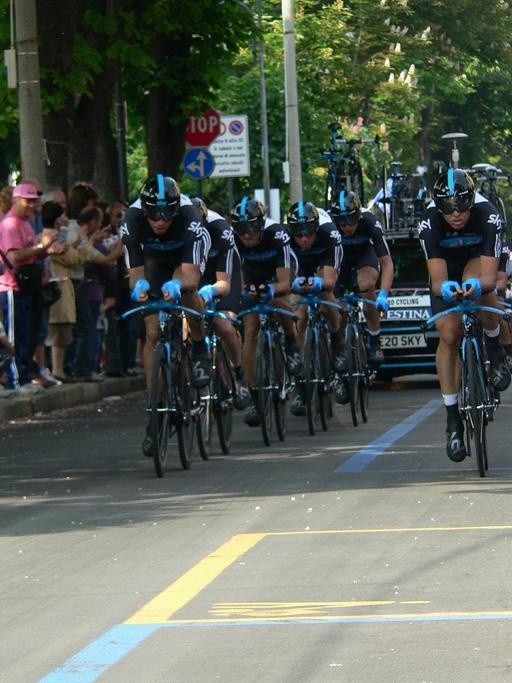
[39,282,62,307]
[15,264,42,291]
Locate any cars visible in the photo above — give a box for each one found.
[346,228,443,381]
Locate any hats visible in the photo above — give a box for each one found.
[12,185,39,200]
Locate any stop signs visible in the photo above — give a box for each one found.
[184,101,220,147]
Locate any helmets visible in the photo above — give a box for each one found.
[189,197,208,218]
[331,189,361,218]
[286,201,319,230]
[433,169,476,213]
[230,198,265,226]
[141,174,181,215]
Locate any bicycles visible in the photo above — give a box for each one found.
[420,283,512,478]
[188,292,237,459]
[289,275,347,435]
[335,290,386,426]
[233,283,299,443]
[120,290,201,476]
[305,122,509,247]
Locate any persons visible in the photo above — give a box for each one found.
[119,173,213,454]
[181,177,512,422]
[1,176,147,399]
[413,165,510,461]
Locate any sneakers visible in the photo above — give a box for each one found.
[447,420,466,462]
[142,330,383,457]
[489,348,511,390]
[0,368,137,397]
